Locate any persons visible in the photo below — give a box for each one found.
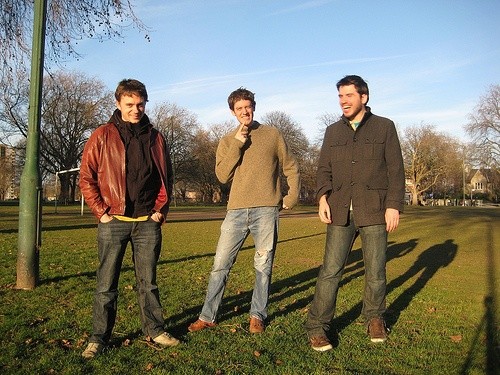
[79,79,180,358]
[188,87,300,333]
[307,74,405,352]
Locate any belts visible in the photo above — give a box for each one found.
[113,215,149,222]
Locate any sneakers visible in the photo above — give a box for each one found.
[308,334,332,351]
[367,317,389,343]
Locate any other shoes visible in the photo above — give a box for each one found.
[81,343,103,359]
[146,332,180,346]
[249,315,265,333]
[187,318,216,333]
